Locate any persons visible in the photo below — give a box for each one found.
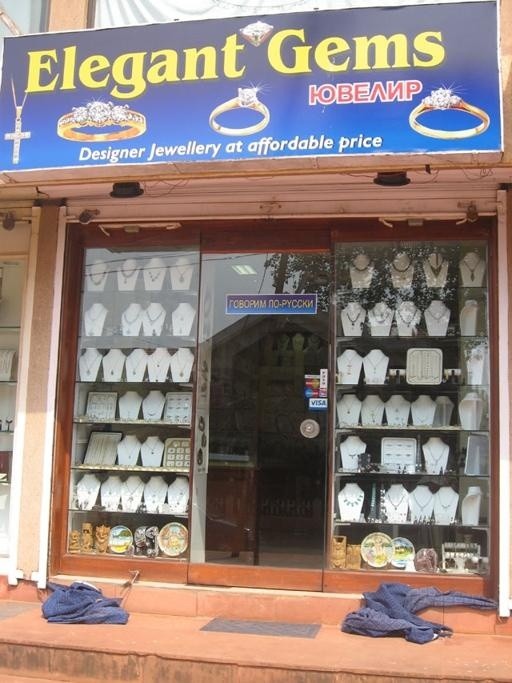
[85,257,196,293]
[78,347,104,382]
[76,473,101,511]
[336,348,363,386]
[335,392,362,428]
[337,483,482,526]
[457,250,486,288]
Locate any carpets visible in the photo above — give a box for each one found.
[199,618,321,638]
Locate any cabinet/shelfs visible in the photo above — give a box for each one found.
[67,290,198,562]
[0,253,31,485]
[331,287,491,577]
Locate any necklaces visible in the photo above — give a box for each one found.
[81,262,195,512]
[339,251,482,512]
[5,77,32,163]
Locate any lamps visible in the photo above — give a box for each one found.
[109,182,144,198]
[373,172,411,187]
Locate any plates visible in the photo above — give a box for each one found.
[361,532,415,568]
[106,525,134,555]
[156,521,188,556]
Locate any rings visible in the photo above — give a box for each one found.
[55,98,145,143]
[407,88,491,141]
[210,87,269,136]
